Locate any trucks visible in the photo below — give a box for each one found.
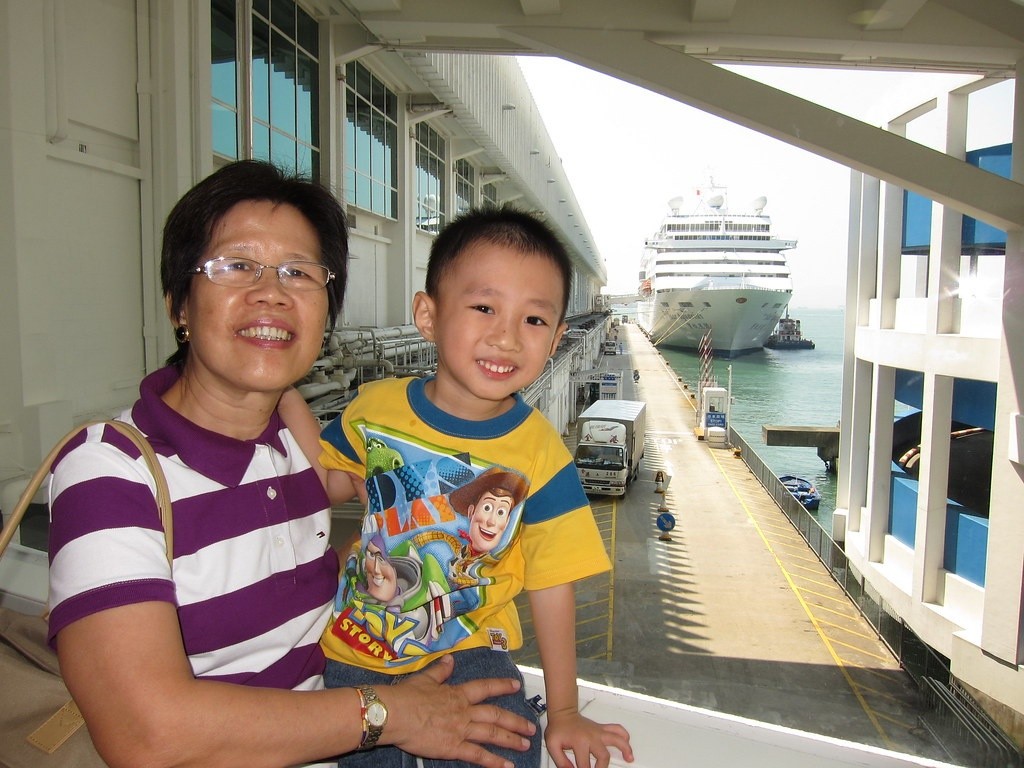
[574,399,646,498]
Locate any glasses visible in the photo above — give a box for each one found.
[190,256,335,291]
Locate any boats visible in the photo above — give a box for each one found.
[765,304,816,349]
[779,475,821,510]
[637,281,651,296]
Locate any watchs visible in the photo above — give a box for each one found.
[352,686,388,751]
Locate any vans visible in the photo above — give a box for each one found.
[605,341,617,354]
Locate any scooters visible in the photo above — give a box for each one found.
[634,375,639,384]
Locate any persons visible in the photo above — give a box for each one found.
[46,161,537,768]
[276,205,636,768]
[619,342,623,356]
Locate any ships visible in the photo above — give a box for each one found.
[635,184,798,358]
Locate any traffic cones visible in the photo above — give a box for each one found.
[657,492,669,511]
[659,529,672,541]
[654,473,664,493]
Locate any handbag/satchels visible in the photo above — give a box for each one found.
[0,419,174,768]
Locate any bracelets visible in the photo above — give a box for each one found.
[356,689,367,748]
